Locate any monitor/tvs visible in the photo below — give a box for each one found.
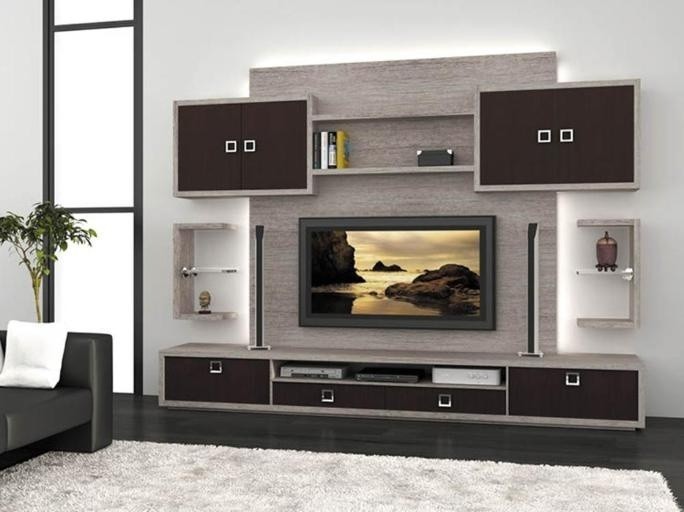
[298,216,496,331]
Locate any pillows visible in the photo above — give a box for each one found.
[1,317,73,393]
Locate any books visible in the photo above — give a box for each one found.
[313,131,350,169]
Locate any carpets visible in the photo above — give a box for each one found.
[0,434,682,512]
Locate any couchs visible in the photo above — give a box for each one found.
[0,322,116,471]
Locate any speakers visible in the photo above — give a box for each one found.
[247,225,271,351]
[517,223,544,357]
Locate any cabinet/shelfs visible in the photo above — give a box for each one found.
[154,340,647,436]
[311,106,473,177]
[170,221,240,323]
[169,93,317,200]
[574,218,642,331]
[473,77,643,194]
